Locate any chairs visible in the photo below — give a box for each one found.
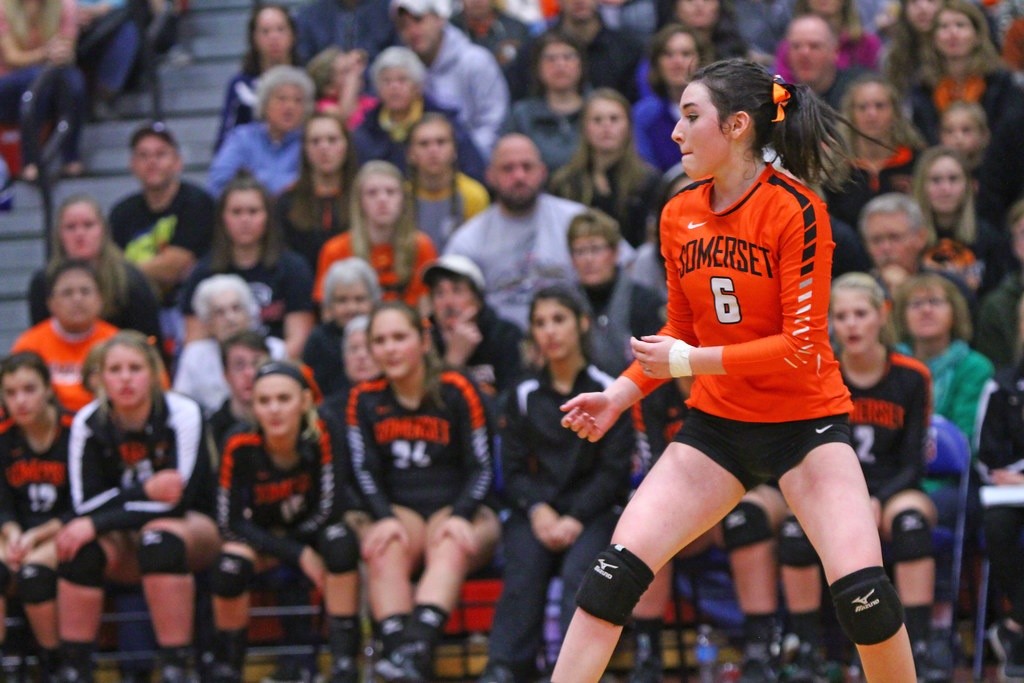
[0,379,1024,683]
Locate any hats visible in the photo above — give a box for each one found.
[388,0,453,21]
[130,123,178,149]
[422,254,488,295]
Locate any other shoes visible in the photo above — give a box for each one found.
[481,626,1023,681]
[373,646,429,683]
[43,651,362,682]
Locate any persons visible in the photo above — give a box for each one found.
[1,0,1023,683]
[550,57,920,683]
